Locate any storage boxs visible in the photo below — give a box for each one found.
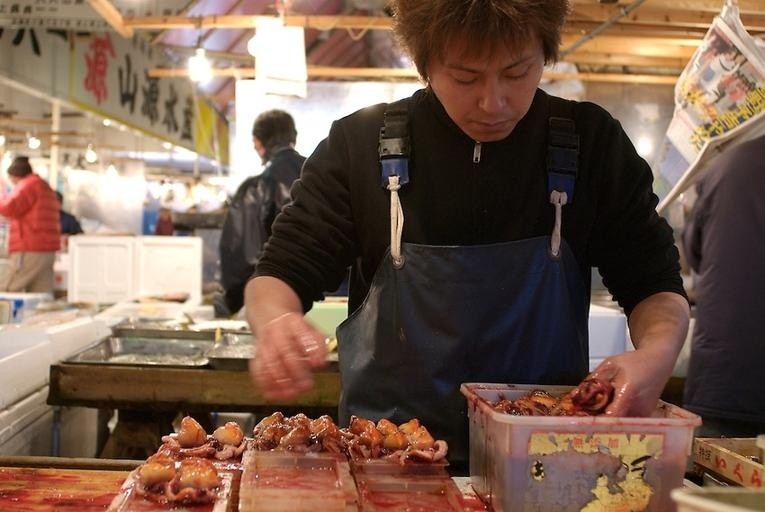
[460,380,704,512]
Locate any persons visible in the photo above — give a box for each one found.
[51,187,87,235]
[683,40,754,127]
[153,207,176,236]
[682,126,765,442]
[242,2,692,434]
[218,109,311,315]
[0,155,63,296]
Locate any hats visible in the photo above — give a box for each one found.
[7,154,33,175]
